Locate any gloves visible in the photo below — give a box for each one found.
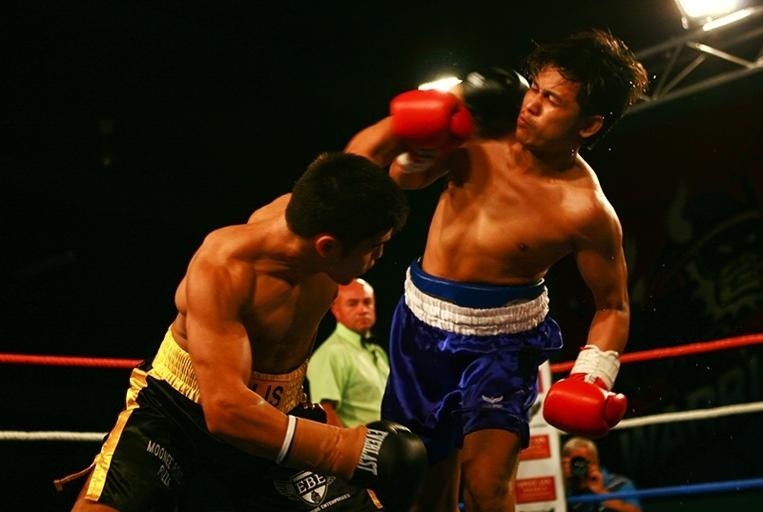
[543,344,628,440]
[455,66,530,139]
[390,89,474,164]
[275,414,430,504]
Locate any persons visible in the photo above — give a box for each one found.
[306,276,391,432]
[382,31,647,511]
[52,67,529,511]
[563,437,640,512]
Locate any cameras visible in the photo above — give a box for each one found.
[570,455,587,474]
